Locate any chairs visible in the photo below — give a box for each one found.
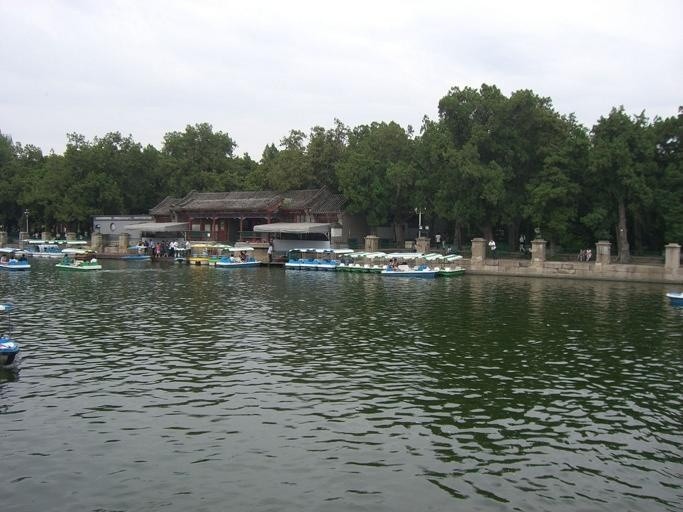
[8,260,27,266]
[289,259,462,273]
[79,261,96,268]
[192,253,254,264]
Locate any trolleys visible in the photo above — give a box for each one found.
[283,248,465,277]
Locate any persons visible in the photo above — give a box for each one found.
[487,238,497,259]
[0,254,8,263]
[8,256,18,263]
[435,232,441,248]
[584,246,592,263]
[266,242,272,262]
[577,247,585,261]
[416,258,426,270]
[230,254,236,263]
[440,232,447,249]
[518,234,525,253]
[135,238,191,258]
[18,253,27,262]
[393,258,399,271]
[386,259,395,271]
[240,253,245,261]
[80,259,89,267]
[60,253,71,266]
[89,256,97,265]
[73,254,84,267]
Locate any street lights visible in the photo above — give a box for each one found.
[415,206,426,238]
[24,208,30,232]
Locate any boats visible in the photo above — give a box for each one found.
[666,291,683,303]
[0,247,31,271]
[174,244,263,267]
[0,304,20,366]
[24,245,102,271]
[120,247,151,261]
